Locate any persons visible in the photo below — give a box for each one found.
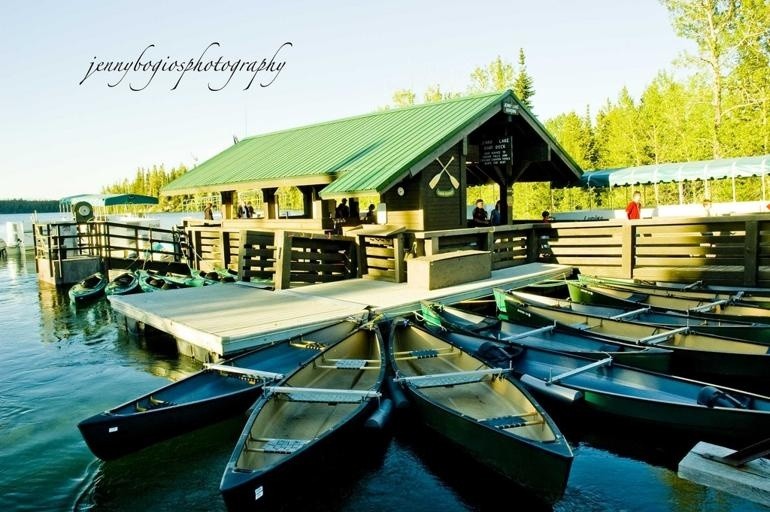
[472,198,489,225]
[337,198,348,216]
[541,211,550,223]
[489,200,501,225]
[625,191,642,220]
[702,198,713,217]
[363,203,376,224]
[203,203,214,220]
[236,200,257,220]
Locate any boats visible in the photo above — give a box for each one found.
[0,239,7,252]
[53,193,160,236]
[550,156,770,222]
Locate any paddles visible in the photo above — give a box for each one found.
[435,157,459,190]
[429,156,454,189]
